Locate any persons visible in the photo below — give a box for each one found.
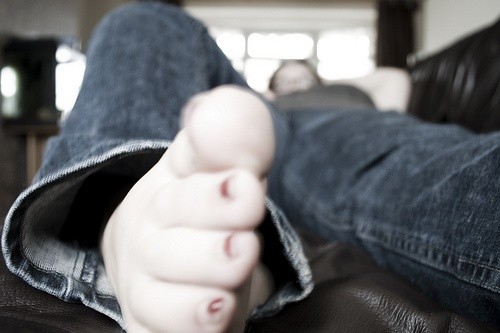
[1,0,500,333]
